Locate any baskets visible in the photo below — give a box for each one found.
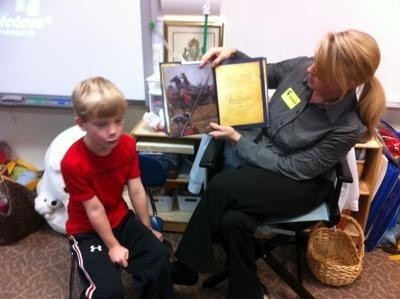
[306,215,365,287]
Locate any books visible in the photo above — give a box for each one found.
[158,55,270,138]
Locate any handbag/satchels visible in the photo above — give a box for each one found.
[0,175,46,245]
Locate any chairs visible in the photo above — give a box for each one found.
[198,124,353,299]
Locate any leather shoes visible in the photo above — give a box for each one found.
[170,261,198,286]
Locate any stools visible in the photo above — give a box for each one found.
[69,251,76,299]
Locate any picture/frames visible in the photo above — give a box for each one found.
[163,21,224,62]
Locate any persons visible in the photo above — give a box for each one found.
[171,29,387,299]
[182,38,205,61]
[61,76,174,299]
[175,112,200,136]
[179,73,190,90]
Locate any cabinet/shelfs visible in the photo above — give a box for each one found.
[131,118,382,240]
